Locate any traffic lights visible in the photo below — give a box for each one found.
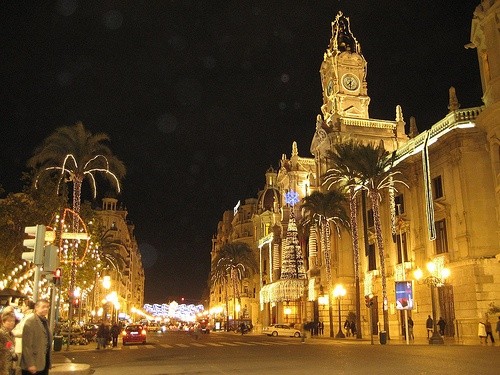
[54,268,62,277]
[21,224,47,265]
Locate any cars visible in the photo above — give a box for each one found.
[123,324,147,344]
[137,318,207,333]
[262,324,301,338]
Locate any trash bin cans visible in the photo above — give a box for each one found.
[380,331,387,345]
[54,336,63,352]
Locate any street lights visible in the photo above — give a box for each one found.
[331,283,347,340]
[414,262,452,344]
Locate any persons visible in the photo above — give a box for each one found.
[304,319,324,337]
[19,300,53,375]
[437,316,445,336]
[344,320,355,337]
[194,323,199,340]
[495,317,500,340]
[95,321,119,349]
[426,316,434,338]
[0,312,17,375]
[406,317,415,340]
[478,320,488,343]
[238,322,253,334]
[484,320,495,343]
